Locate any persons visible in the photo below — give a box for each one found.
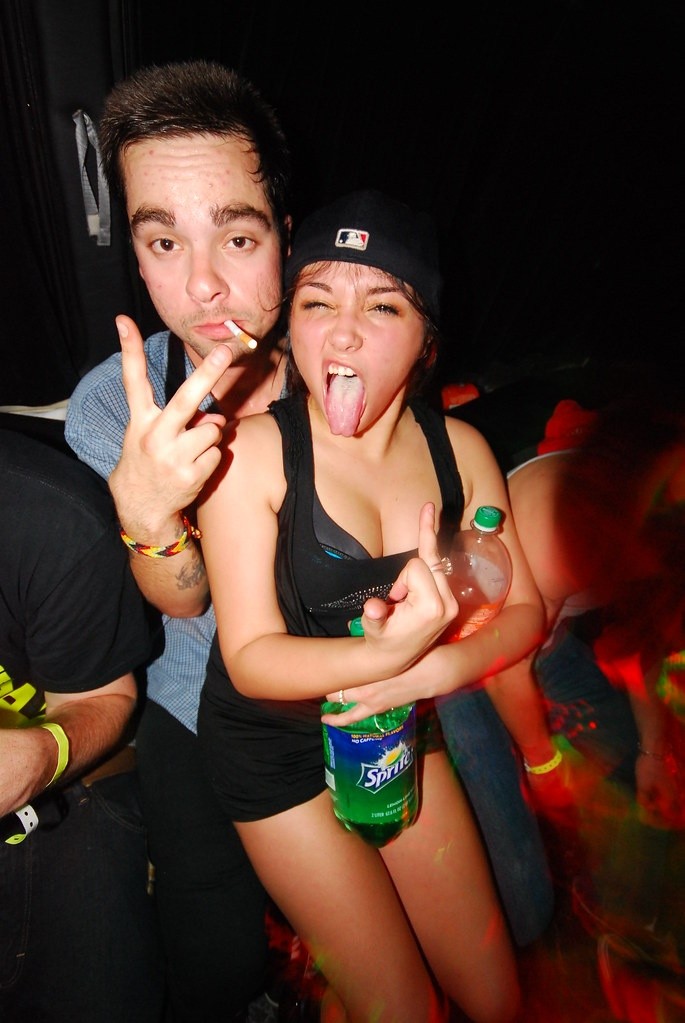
[491,385,685,838]
[61,61,553,957]
[196,190,546,1023]
[0,409,267,1023]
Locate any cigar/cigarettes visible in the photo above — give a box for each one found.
[225,320,257,349]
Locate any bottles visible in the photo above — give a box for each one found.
[320,617,419,848]
[437,505,513,644]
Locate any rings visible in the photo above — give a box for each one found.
[339,690,348,706]
[429,558,453,577]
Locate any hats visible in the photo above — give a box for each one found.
[286,189,443,330]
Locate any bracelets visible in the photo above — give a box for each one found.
[119,511,202,559]
[40,723,69,787]
[524,747,561,775]
